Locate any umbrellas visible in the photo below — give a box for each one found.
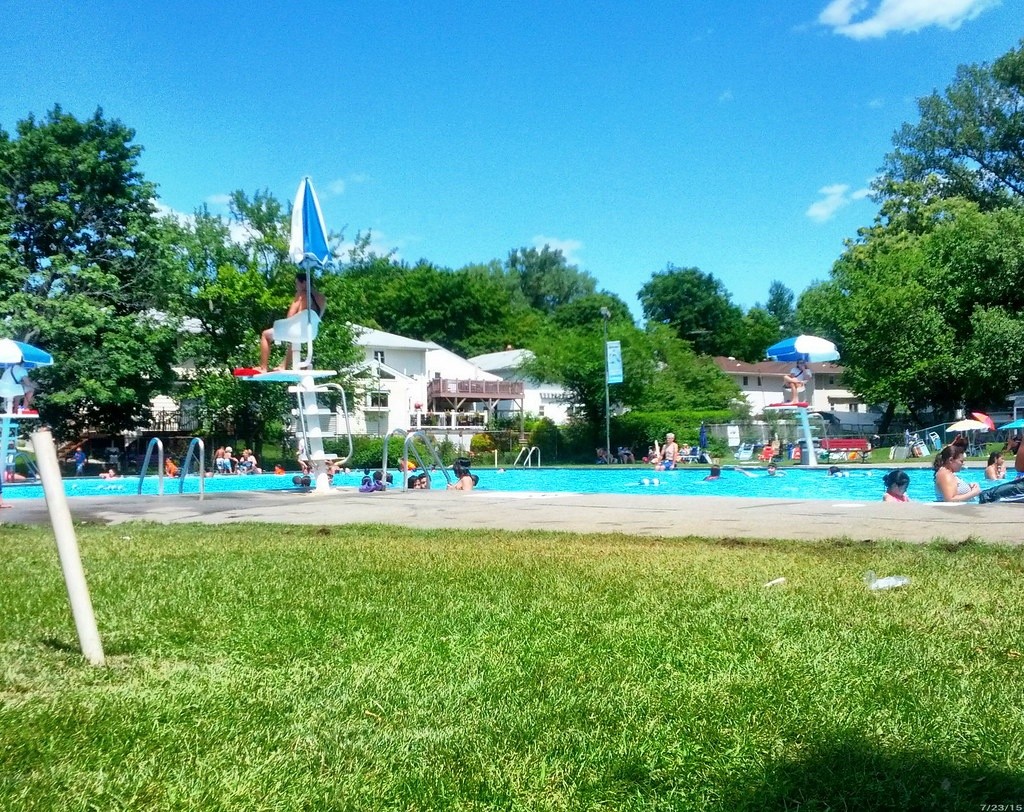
[0,337,54,410]
[287,177,333,366]
[766,334,840,362]
[700,422,708,453]
[998,419,1024,429]
[946,419,989,454]
[972,412,995,433]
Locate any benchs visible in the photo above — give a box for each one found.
[822,439,872,463]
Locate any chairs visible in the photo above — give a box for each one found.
[758,445,773,462]
[618,447,630,465]
[927,431,1018,458]
[596,447,613,464]
[735,442,754,461]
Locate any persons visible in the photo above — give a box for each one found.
[597,432,691,471]
[909,441,922,457]
[883,470,912,503]
[0,474,11,508]
[363,457,478,491]
[703,465,720,480]
[768,463,776,475]
[984,435,1024,479]
[165,457,179,478]
[252,274,327,373]
[829,467,840,476]
[784,359,812,403]
[100,469,117,478]
[214,446,345,487]
[934,433,981,502]
[2,361,40,412]
[71,448,86,477]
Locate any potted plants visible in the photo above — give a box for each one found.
[711,446,720,464]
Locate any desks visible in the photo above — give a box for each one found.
[676,456,699,464]
